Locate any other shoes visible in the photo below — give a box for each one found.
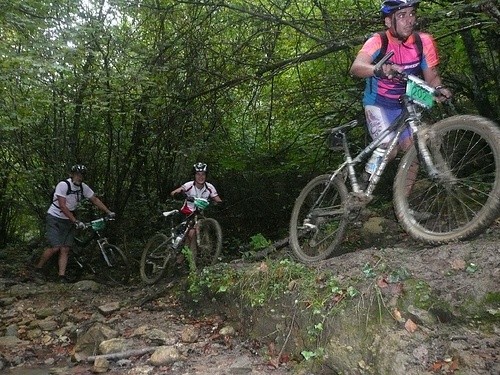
[32,272,45,285]
[56,277,69,285]
[357,209,376,216]
[174,264,191,274]
[393,208,433,222]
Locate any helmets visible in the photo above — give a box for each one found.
[193,162,207,173]
[380,0,422,20]
[70,164,88,175]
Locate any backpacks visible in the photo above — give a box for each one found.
[50,179,85,204]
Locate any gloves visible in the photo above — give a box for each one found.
[106,211,115,217]
[215,201,226,211]
[173,189,188,201]
[73,219,86,231]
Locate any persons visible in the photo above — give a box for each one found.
[33,163,115,283]
[171,161,222,273]
[351,0,451,221]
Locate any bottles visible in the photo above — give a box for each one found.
[171,234,184,250]
[364,143,388,175]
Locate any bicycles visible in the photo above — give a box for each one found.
[288,50,500,263]
[52,214,130,286]
[139,190,222,285]
